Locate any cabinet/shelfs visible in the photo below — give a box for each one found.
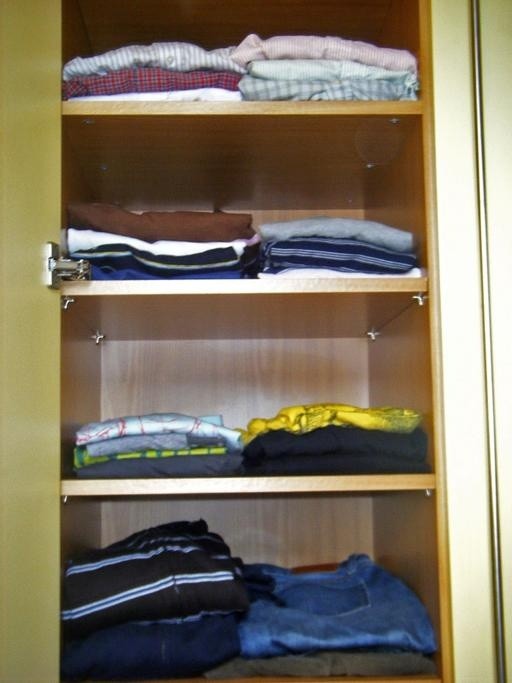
[3,0,492,682]
